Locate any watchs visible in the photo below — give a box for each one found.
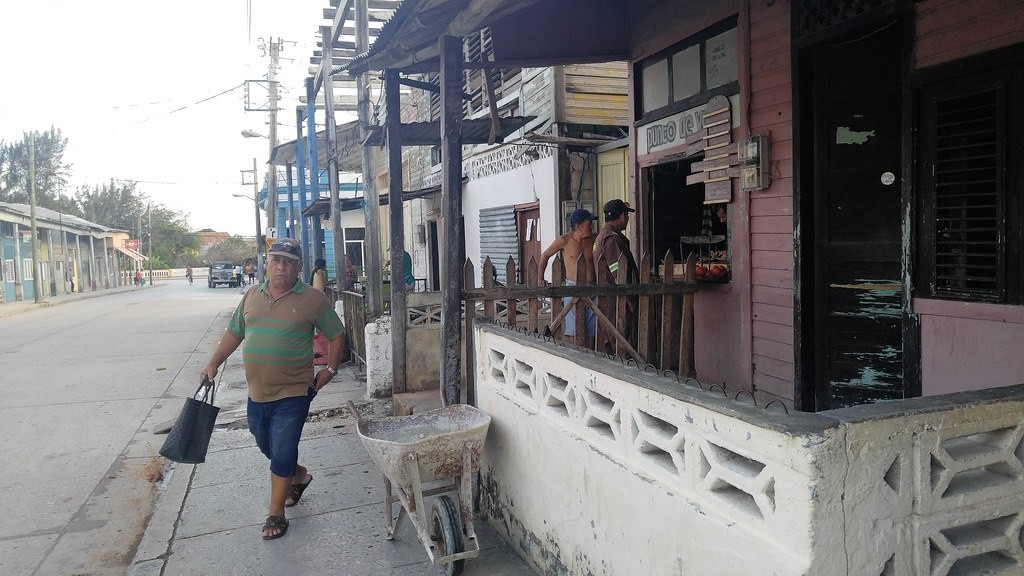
[327,365,338,376]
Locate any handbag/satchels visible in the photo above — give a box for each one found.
[159,374,220,464]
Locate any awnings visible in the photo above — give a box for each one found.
[117,247,148,261]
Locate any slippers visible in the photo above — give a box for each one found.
[284,474,313,507]
[262,516,289,540]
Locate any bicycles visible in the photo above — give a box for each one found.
[187,274,192,285]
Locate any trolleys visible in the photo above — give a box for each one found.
[345,398,492,576]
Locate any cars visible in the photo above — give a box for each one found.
[207,261,239,289]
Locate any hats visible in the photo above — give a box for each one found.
[267,237,303,261]
[603,199,636,212]
[569,209,599,222]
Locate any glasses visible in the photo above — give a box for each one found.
[268,243,299,255]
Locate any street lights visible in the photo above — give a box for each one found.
[232,193,264,284]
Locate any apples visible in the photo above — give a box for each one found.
[695,264,727,279]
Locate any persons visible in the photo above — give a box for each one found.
[185,266,194,282]
[537,209,599,349]
[232,261,255,286]
[712,204,727,260]
[309,259,326,336]
[593,199,640,359]
[384,244,415,317]
[66,267,75,293]
[135,268,145,287]
[199,238,345,540]
[345,253,358,291]
